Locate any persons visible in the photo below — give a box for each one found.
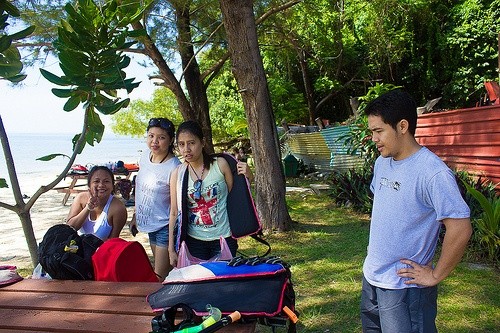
[66,166,127,242]
[231,146,245,160]
[168,120,254,267]
[360,88,473,333]
[130,117,182,282]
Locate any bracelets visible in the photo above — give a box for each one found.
[85,204,94,211]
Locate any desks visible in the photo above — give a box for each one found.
[0,279,256,333]
[62,169,139,206]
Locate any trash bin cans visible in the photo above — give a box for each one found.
[283,154,298,177]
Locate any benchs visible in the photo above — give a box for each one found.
[56,189,88,193]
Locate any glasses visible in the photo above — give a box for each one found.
[193,179,202,200]
[148,118,173,130]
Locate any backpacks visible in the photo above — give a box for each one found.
[90,237,161,283]
[38,224,104,282]
[146,253,292,318]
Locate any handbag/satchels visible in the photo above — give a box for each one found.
[177,235,235,269]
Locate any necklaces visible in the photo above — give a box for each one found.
[190,164,205,179]
[150,151,169,163]
